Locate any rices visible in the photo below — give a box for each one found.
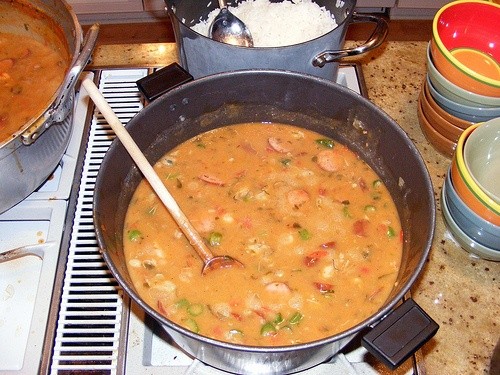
[184,1,348,47]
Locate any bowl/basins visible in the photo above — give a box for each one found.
[419,0,500,262]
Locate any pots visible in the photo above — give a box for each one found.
[93,61,439,375]
[0,0,99,213]
[163,0,388,83]
